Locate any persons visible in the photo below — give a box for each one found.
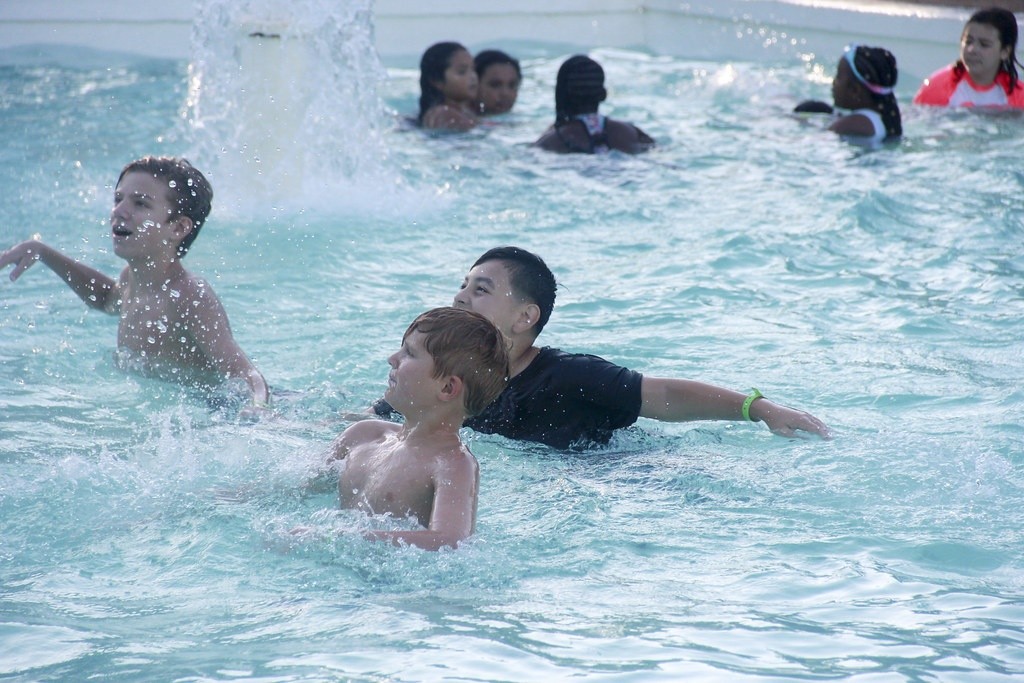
[291,308,513,554]
[913,8,1024,109]
[0,157,268,416]
[363,246,830,446]
[827,44,902,143]
[416,42,479,132]
[474,49,522,116]
[532,55,654,155]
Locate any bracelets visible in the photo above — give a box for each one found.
[741,386,767,424]
